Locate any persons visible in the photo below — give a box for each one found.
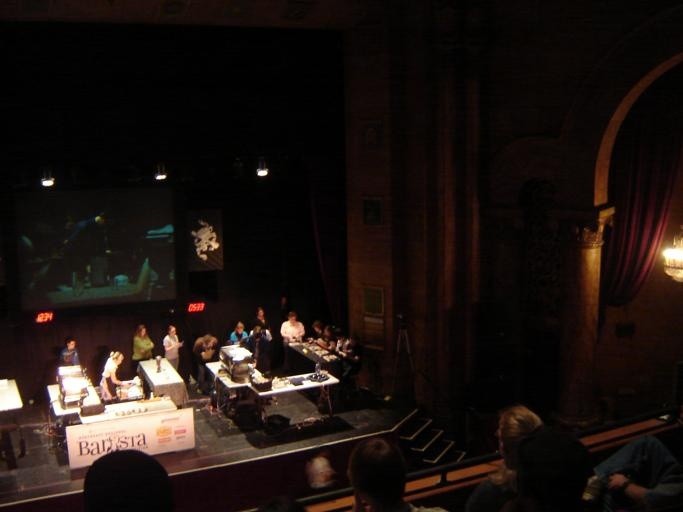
[132,325,154,378]
[100,352,131,403]
[163,326,182,370]
[465,404,683,512]
[83,451,177,510]
[193,335,218,394]
[346,437,446,511]
[60,338,80,367]
[231,308,358,378]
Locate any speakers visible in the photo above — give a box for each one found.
[179,208,225,272]
[444,409,485,451]
[576,414,611,435]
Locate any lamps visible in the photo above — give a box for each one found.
[661,224,683,284]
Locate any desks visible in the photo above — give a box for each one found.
[46,378,102,448]
[205,359,267,422]
[288,339,355,367]
[1,379,31,459]
[249,369,340,418]
[136,357,191,413]
